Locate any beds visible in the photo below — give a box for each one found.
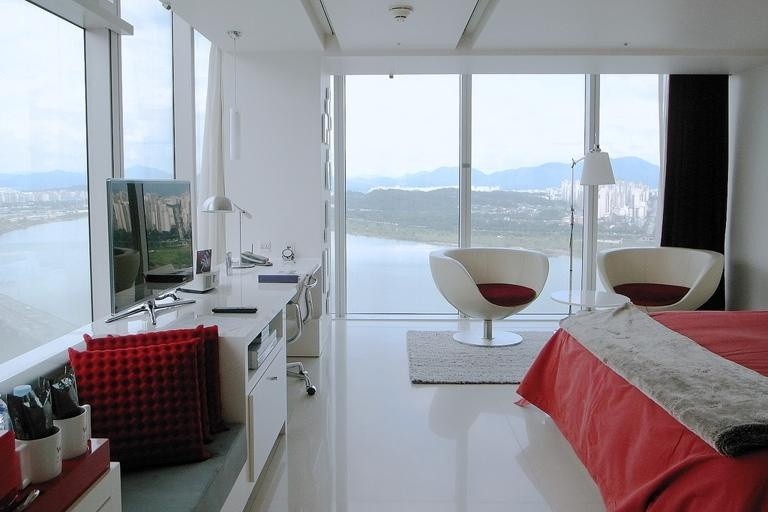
[513,310,768,511]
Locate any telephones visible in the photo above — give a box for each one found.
[240,243,269,264]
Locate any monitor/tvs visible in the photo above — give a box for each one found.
[104,177,197,325]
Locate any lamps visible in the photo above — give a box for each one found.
[560,142,614,325]
[201,195,258,270]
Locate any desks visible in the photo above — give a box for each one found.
[107,257,327,486]
[551,290,628,325]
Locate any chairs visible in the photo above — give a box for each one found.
[283,266,326,395]
[595,248,724,315]
[429,245,549,346]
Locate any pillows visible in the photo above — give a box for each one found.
[70,323,218,477]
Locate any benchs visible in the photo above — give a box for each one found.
[118,422,251,512]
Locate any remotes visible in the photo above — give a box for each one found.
[212,306,258,313]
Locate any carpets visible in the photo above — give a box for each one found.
[406,327,556,382]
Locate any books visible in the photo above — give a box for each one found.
[257,273,301,283]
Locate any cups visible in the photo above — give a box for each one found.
[45,404,93,460]
[12,425,62,490]
